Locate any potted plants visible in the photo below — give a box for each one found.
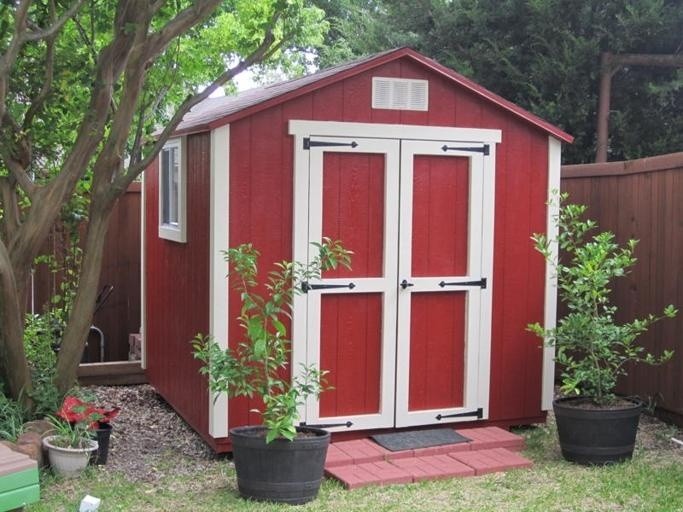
[522,190,677,465]
[189,233,355,504]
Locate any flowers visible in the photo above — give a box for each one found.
[47,396,119,443]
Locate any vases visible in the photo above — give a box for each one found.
[43,423,113,479]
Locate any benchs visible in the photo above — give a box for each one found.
[0,439,40,512]
